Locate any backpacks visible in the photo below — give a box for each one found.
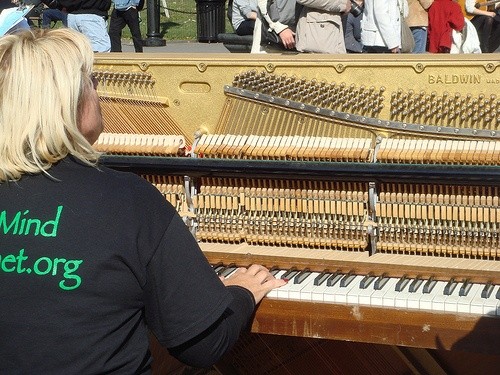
[266,0,297,27]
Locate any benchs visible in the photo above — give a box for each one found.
[217,33,254,52]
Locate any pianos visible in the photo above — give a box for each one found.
[88,53,500,358]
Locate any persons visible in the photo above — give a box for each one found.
[108,0,146,53]
[0,23,288,375]
[67,0,112,54]
[227,0,500,53]
[0,0,69,33]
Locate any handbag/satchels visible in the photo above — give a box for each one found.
[400,13,415,53]
